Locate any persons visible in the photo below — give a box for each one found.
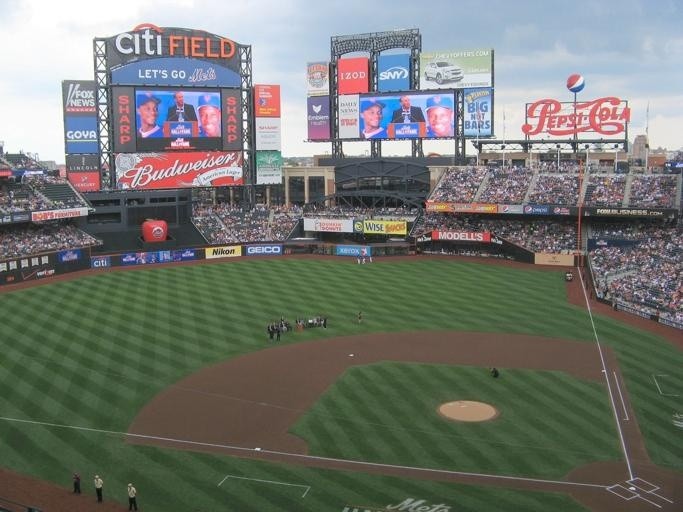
[136,94,163,138]
[95,475,103,503]
[73,473,81,494]
[167,92,196,121]
[359,101,387,139]
[491,368,500,377]
[391,96,425,122]
[127,483,137,510]
[424,96,453,137]
[197,95,221,135]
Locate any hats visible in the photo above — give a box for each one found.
[135,93,160,108]
[360,99,386,112]
[425,96,452,112]
[197,95,220,110]
